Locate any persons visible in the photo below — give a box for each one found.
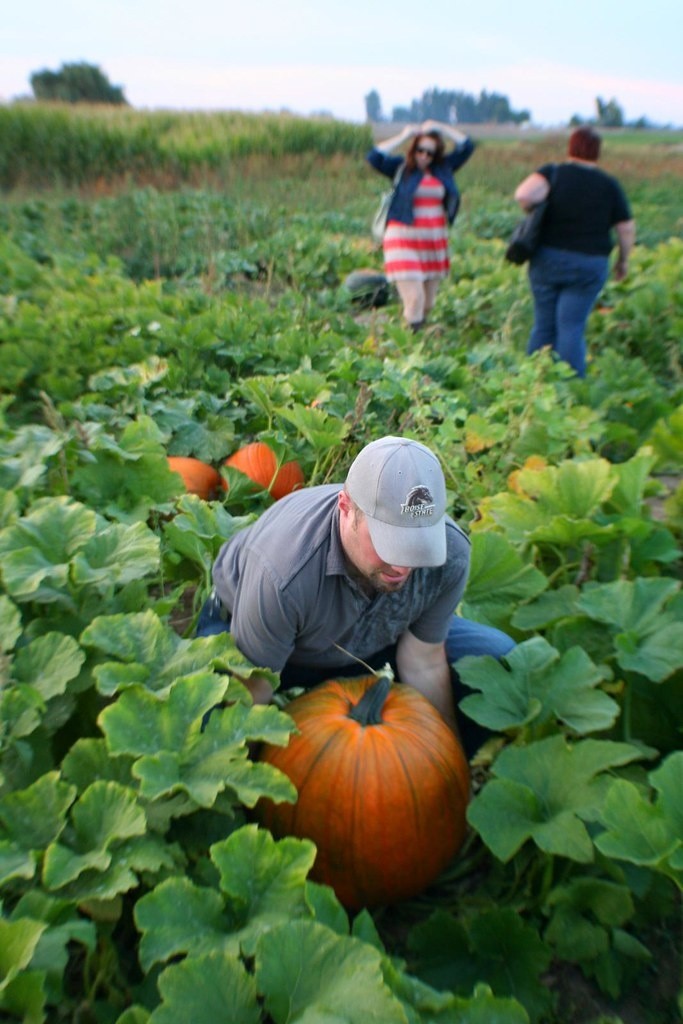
[514,126,635,380]
[191,435,532,891]
[366,119,477,338]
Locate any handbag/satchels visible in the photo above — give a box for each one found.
[507,162,559,265]
[371,161,407,237]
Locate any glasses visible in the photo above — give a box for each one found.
[415,145,435,157]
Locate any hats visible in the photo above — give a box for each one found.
[346,436,446,567]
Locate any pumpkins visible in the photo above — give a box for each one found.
[167,456,221,503]
[221,442,304,501]
[342,268,389,310]
[248,674,470,912]
